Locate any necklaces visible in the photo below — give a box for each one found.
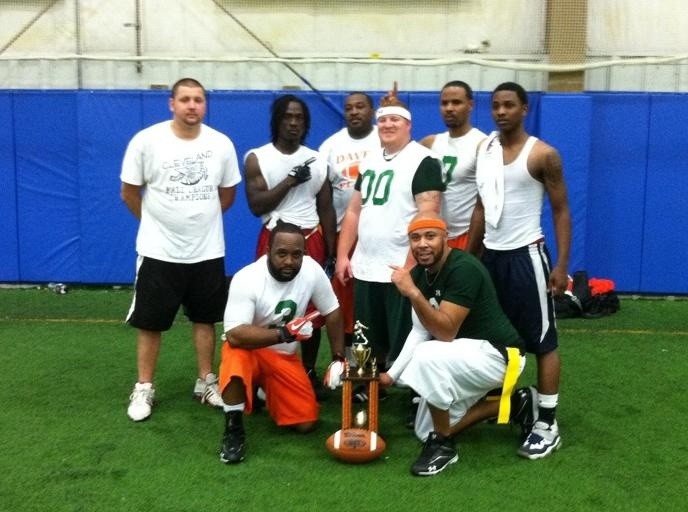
[383,141,411,161]
[425,268,440,286]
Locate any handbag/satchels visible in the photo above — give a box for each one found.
[553,270,621,320]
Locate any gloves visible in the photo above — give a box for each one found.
[287,155,318,185]
[322,351,347,390]
[278,310,320,345]
[321,253,338,283]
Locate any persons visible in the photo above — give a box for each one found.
[378,210,539,476]
[120,79,242,422]
[318,91,382,368]
[464,82,571,461]
[335,95,444,429]
[218,223,345,465]
[419,81,488,250]
[242,93,337,405]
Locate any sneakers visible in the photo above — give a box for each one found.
[514,416,562,461]
[512,385,540,445]
[410,431,459,476]
[193,372,224,409]
[219,415,246,463]
[126,380,155,422]
[337,384,389,404]
[406,389,420,429]
[305,367,328,401]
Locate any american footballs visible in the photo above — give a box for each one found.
[325,427,386,464]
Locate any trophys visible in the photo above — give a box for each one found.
[340,319,381,433]
